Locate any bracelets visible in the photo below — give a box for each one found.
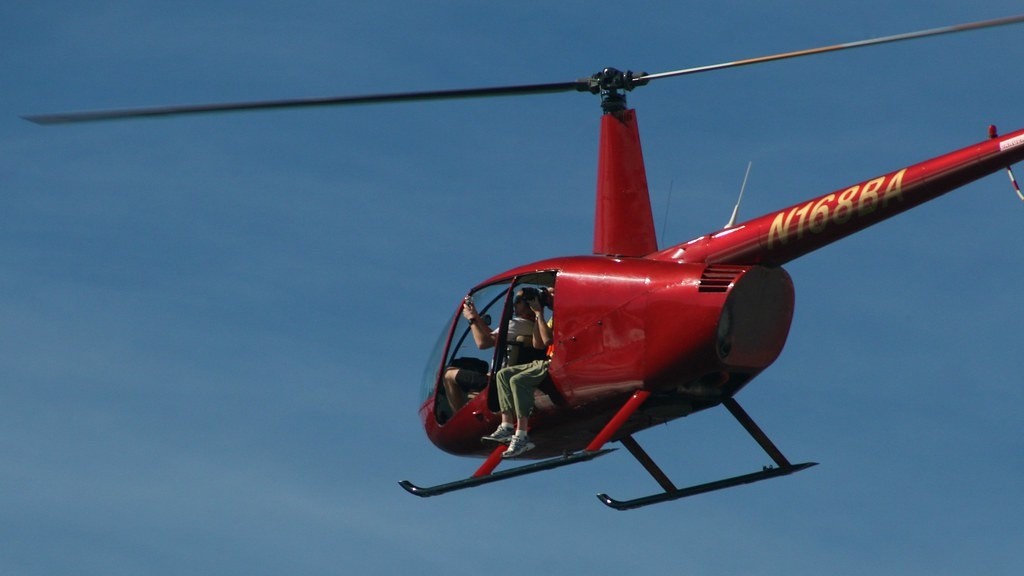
[536,311,542,318]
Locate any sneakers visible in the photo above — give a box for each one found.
[480,424,514,443]
[501,435,535,459]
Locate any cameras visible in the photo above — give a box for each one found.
[521,288,550,306]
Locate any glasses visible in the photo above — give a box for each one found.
[514,294,521,304]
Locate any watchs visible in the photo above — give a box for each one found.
[468,318,475,324]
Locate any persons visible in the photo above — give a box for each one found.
[481,285,554,459]
[442,288,543,414]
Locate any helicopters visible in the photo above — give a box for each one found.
[19,15,1024,511]
